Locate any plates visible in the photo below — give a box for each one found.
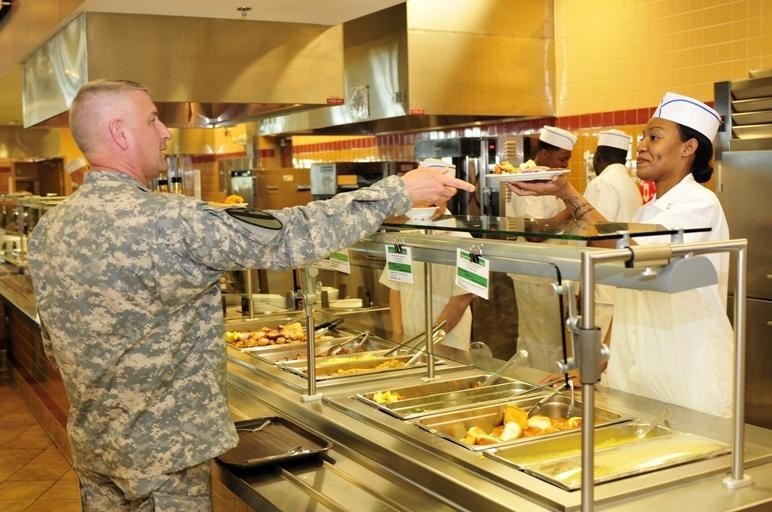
[223,203,249,209]
[252,293,287,314]
[322,286,363,309]
[485,168,571,183]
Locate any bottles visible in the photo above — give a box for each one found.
[156,177,183,194]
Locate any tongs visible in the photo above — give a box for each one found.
[304,318,447,356]
[527,379,575,421]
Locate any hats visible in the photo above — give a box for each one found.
[538,125,577,151]
[597,129,632,151]
[652,91,721,143]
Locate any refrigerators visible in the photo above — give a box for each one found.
[716,77,772,433]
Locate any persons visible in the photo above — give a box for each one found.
[582,132,643,348]
[510,125,577,375]
[28,80,475,512]
[507,92,736,419]
[378,205,474,353]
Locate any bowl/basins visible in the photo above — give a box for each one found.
[404,207,439,222]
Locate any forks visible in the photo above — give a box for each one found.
[236,420,271,433]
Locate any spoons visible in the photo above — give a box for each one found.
[246,446,311,465]
